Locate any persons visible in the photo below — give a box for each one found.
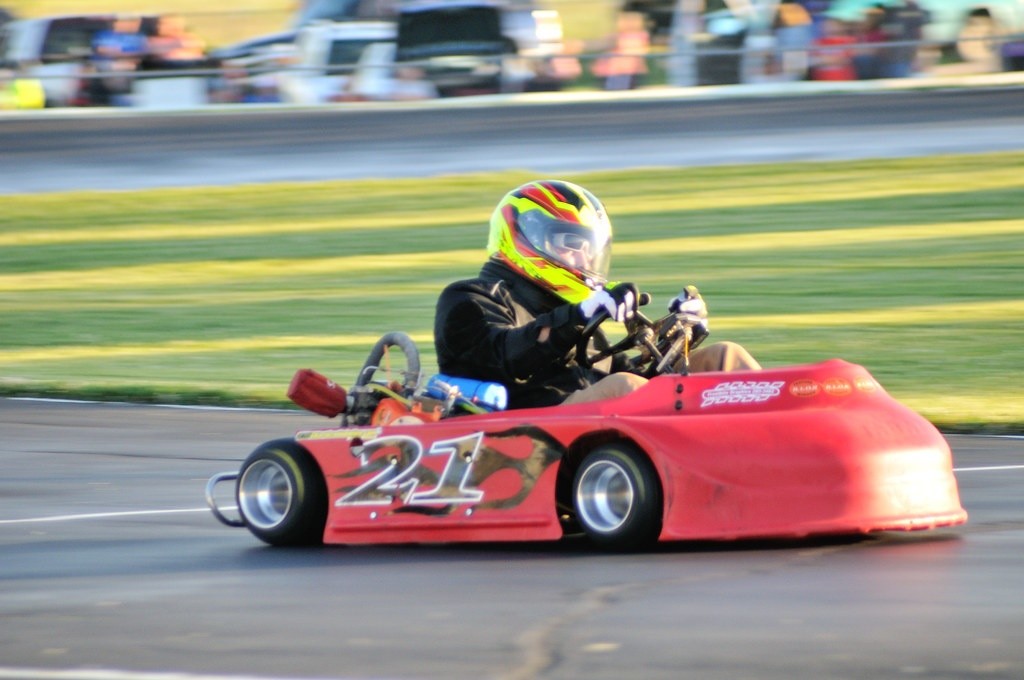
[0,0,1024,111]
[435,179,764,410]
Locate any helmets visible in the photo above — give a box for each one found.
[486,179,614,303]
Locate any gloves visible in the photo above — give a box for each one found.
[579,282,639,322]
[668,285,709,351]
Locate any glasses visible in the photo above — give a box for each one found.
[552,233,597,261]
